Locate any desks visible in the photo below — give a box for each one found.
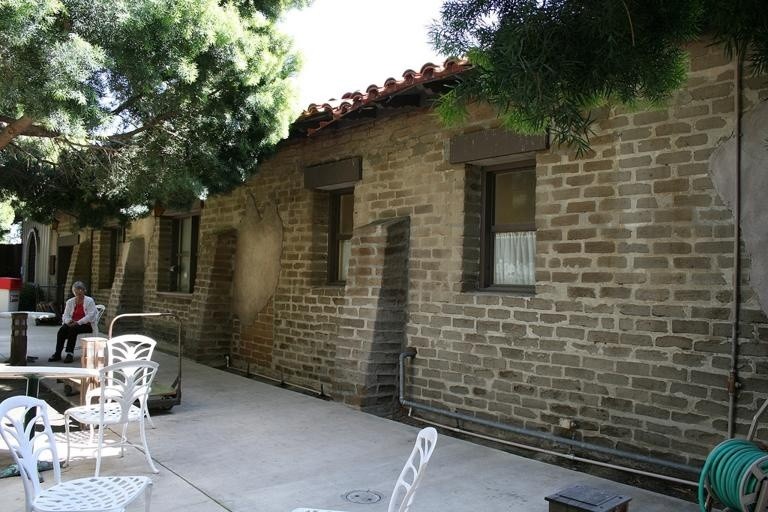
[0,365,105,485]
[0,310,57,365]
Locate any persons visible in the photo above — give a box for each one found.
[48,282,98,363]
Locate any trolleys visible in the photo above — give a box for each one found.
[55,312,183,410]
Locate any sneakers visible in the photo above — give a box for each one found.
[64,354,73,363]
[48,352,61,362]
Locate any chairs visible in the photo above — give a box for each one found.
[83,333,158,445]
[289,424,439,512]
[62,359,162,480]
[63,304,107,350]
[0,394,155,510]
